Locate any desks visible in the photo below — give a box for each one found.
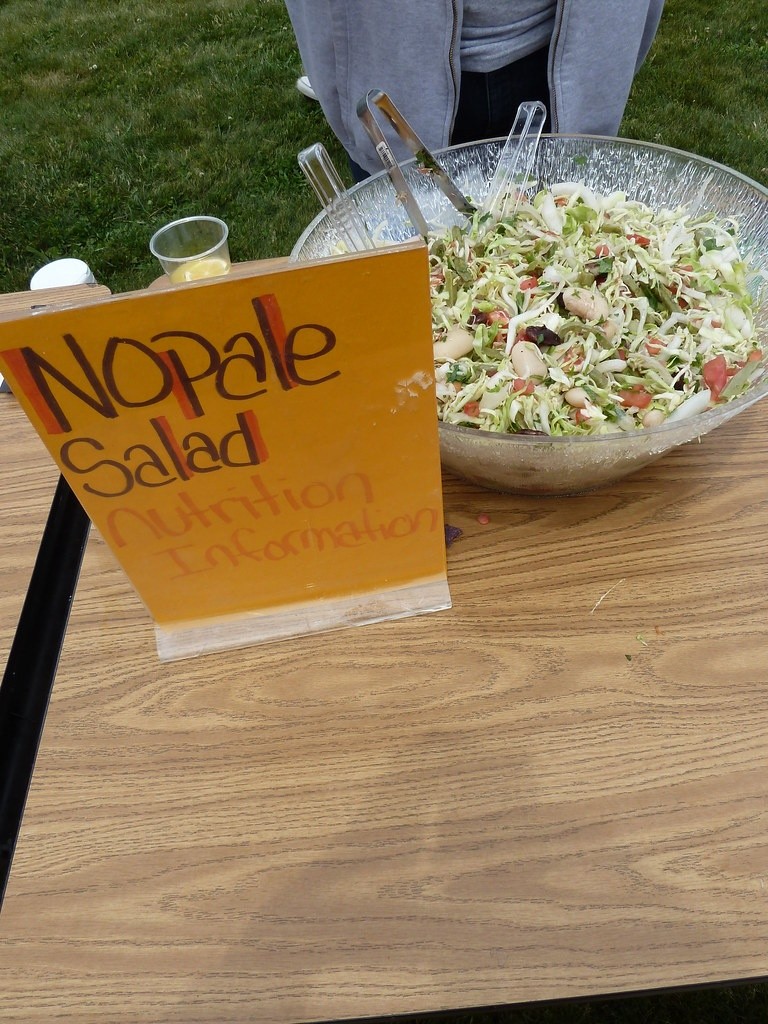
[0,251,768,1024]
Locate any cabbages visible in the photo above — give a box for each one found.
[372,179,764,437]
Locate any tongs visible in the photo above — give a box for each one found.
[296,141,375,253]
[470,100,548,253]
[355,88,490,264]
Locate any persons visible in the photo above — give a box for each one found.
[282,0,665,189]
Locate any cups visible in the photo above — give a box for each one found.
[149,216,234,286]
[31,258,98,290]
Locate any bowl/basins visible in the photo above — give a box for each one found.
[288,133,768,499]
